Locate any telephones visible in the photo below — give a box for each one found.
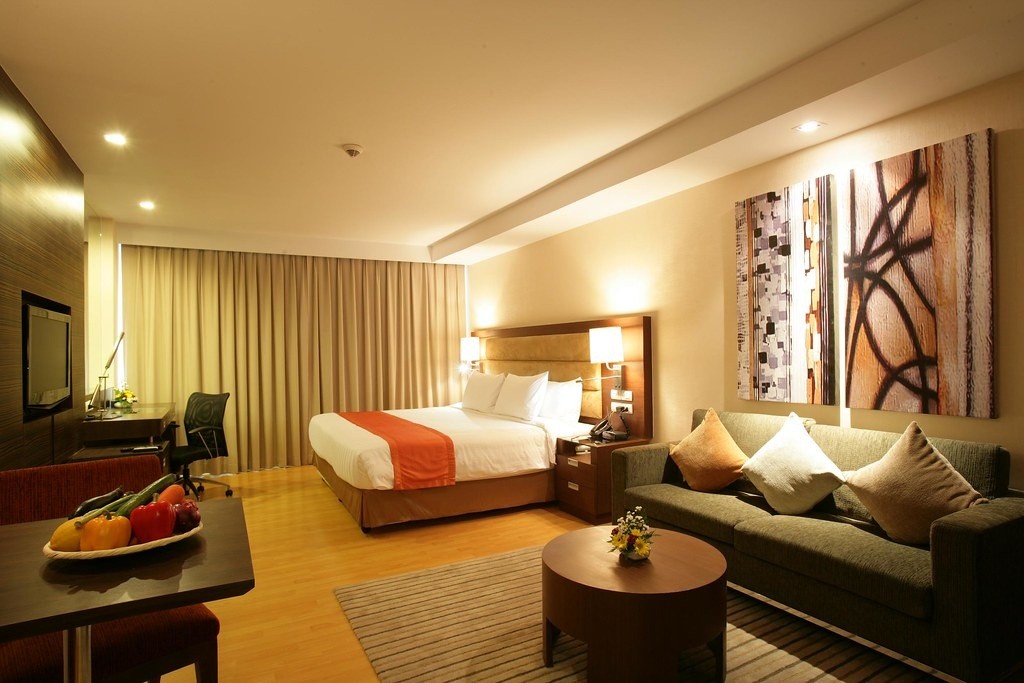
[589,418,611,440]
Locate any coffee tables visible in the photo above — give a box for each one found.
[542,526,727,683]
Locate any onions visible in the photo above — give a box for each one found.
[174,500,202,532]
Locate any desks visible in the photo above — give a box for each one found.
[0,496,255,643]
[68,403,174,475]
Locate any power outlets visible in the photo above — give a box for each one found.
[611,402,633,415]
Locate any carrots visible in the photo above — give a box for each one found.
[129,484,185,544]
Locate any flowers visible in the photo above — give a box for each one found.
[605,506,662,556]
[114,384,138,414]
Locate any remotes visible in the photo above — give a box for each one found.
[133,446,158,451]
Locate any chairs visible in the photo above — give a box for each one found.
[169,392,231,501]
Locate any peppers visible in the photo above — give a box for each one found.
[49,471,181,551]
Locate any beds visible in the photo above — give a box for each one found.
[308,316,651,534]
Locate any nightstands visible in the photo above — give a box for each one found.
[555,432,649,524]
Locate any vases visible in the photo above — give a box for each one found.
[114,402,133,409]
[623,551,650,561]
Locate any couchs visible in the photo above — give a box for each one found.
[612,409,1024,683]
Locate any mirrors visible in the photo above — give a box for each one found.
[21,288,74,423]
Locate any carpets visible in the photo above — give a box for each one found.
[332,544,945,683]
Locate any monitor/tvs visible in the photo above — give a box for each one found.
[22,304,71,410]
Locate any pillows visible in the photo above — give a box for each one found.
[504,377,582,434]
[741,411,846,517]
[493,371,549,420]
[668,408,752,492]
[462,370,506,414]
[845,421,990,545]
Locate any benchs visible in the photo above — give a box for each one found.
[0,454,220,683]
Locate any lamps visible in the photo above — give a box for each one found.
[460,337,482,374]
[588,326,625,388]
